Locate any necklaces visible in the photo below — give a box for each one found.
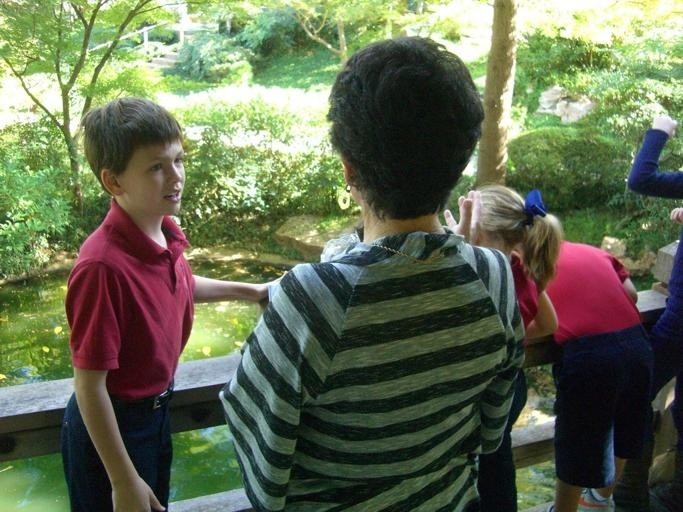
[362,238,451,267]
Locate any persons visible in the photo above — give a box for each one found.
[545,238,655,512]
[475,184,563,511]
[217,31,529,512]
[624,112,683,457]
[57,96,288,512]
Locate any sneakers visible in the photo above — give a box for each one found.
[577,487,616,512]
